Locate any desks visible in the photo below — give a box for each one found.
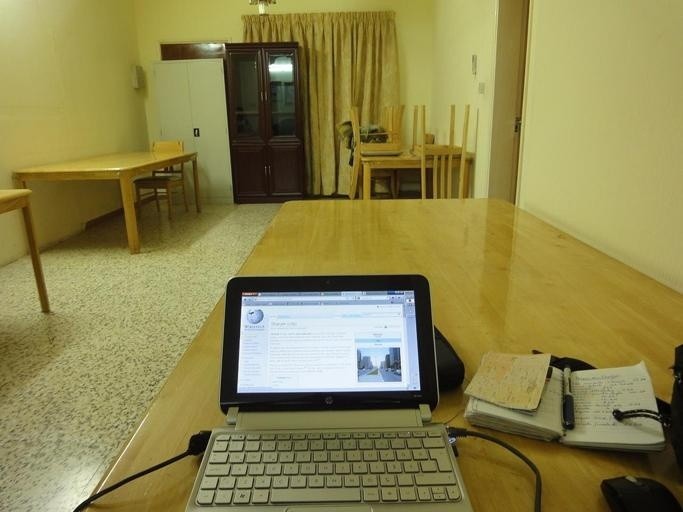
[76,198,681,512]
[10,149,202,254]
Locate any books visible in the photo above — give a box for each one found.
[462,349,667,451]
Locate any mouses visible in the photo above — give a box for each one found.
[601,475,683,512]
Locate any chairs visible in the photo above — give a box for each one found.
[132,141,188,220]
[347,104,474,200]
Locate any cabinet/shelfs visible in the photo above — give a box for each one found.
[150,41,313,204]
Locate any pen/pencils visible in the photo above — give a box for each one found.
[561,361,575,429]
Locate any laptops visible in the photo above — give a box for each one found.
[185,274,472,512]
